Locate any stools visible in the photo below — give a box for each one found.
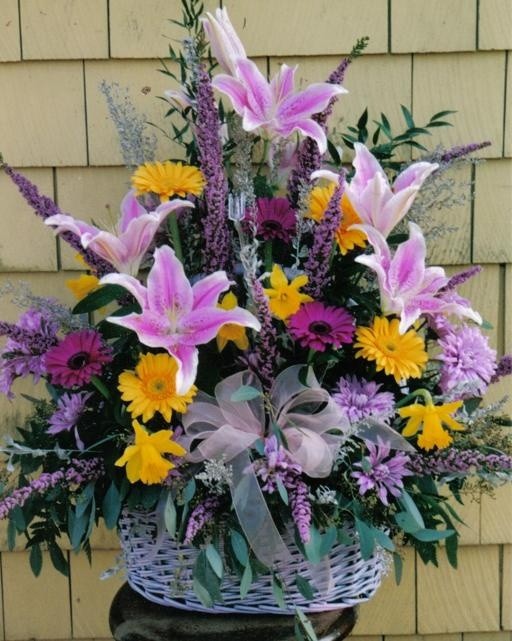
[109,580,359,641]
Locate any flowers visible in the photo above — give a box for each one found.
[0,0,512,641]
